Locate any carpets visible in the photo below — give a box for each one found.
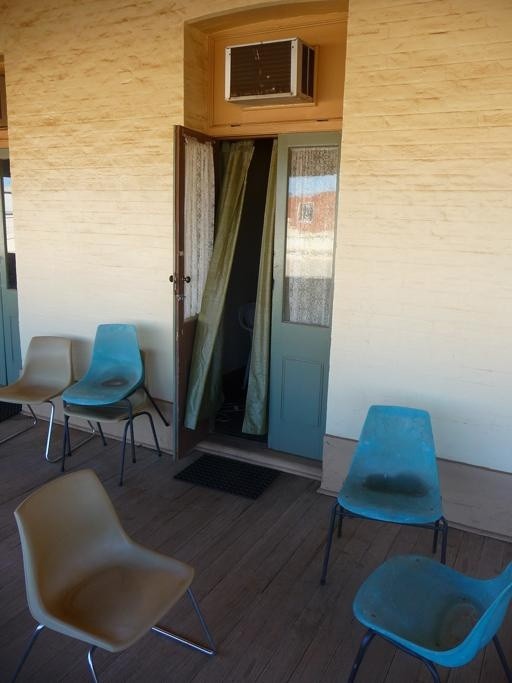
[174,453,284,500]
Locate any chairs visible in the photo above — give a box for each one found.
[12,464,220,682]
[1,321,171,487]
[348,554,511,683]
[319,405,449,586]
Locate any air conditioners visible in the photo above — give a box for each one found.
[223,35,313,109]
